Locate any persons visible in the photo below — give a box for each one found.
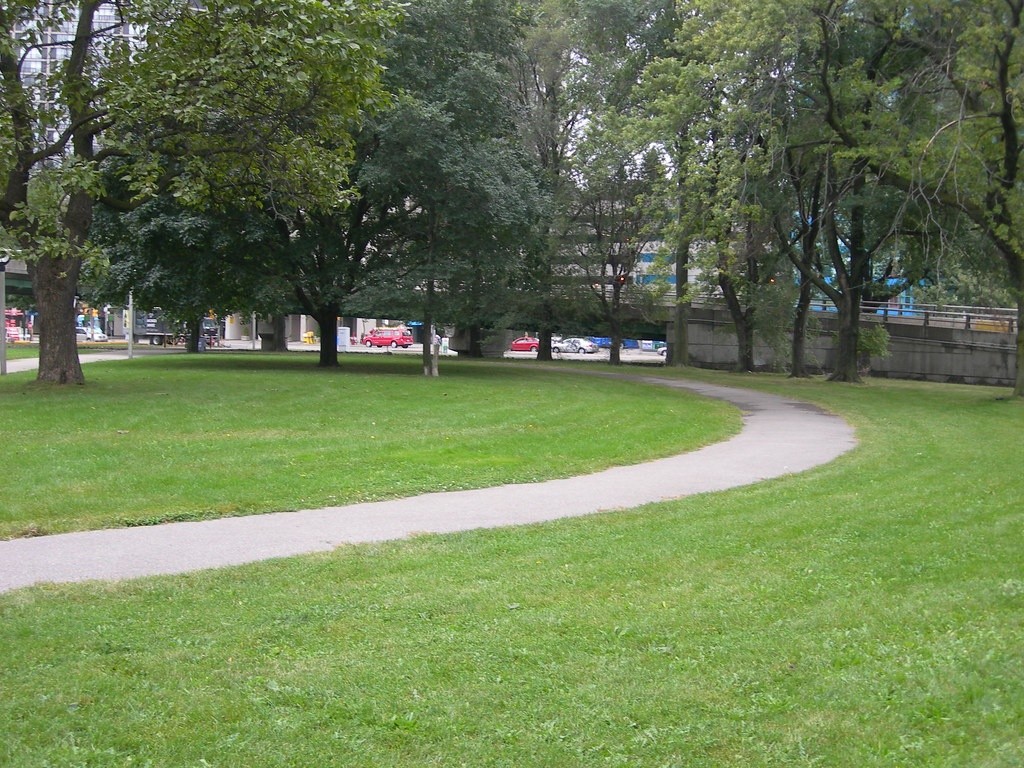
[28,320,34,341]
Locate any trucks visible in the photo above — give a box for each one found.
[133,306,220,345]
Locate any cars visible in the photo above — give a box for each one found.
[657,347,668,357]
[75,327,108,342]
[551,338,599,354]
[508,337,540,352]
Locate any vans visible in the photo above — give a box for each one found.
[362,328,413,349]
